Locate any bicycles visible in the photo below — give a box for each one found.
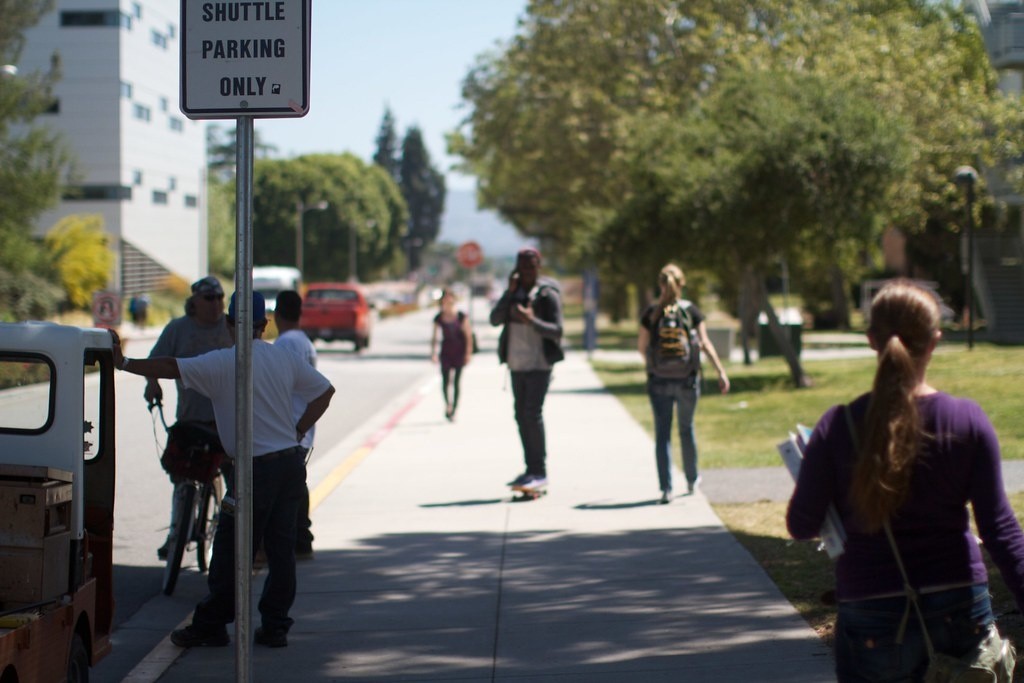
[147,389,223,597]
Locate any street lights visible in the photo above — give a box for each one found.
[955,168,975,349]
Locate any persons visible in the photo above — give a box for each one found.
[107,276,335,648]
[431,287,472,420]
[490,247,564,490]
[786,285,1024,683]
[639,263,731,504]
[127,290,147,327]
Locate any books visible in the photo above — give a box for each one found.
[778,425,849,561]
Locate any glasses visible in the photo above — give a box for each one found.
[204,295,224,301]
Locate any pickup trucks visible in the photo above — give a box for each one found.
[298,285,376,352]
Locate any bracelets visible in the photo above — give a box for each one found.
[117,356,129,371]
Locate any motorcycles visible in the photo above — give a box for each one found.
[0,322,116,683]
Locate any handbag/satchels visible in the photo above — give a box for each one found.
[923,624,1017,683]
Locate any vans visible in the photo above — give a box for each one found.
[251,266,302,312]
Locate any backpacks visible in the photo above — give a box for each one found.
[647,299,704,379]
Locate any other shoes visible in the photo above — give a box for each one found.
[508,471,547,491]
[171,625,231,647]
[293,542,315,561]
[255,626,288,648]
[158,538,169,556]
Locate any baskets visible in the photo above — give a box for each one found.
[160,432,224,484]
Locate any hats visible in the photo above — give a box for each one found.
[229,290,265,320]
[191,276,224,295]
